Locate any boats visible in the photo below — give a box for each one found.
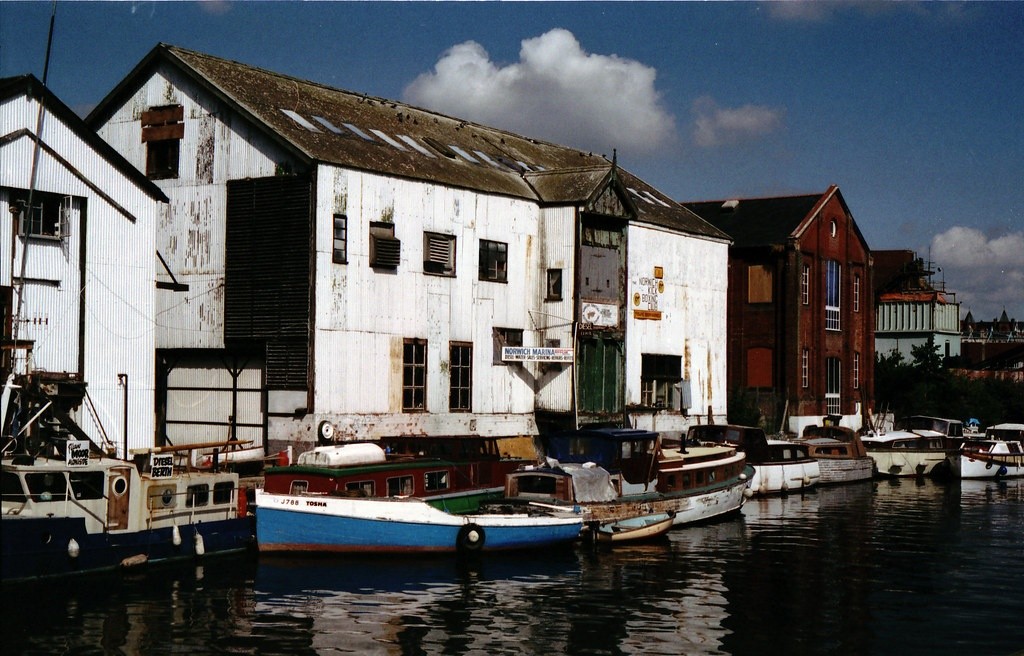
[594,510,679,542]
[680,424,822,495]
[481,429,757,523]
[0,370,255,578]
[799,416,1024,482]
[255,422,584,553]
